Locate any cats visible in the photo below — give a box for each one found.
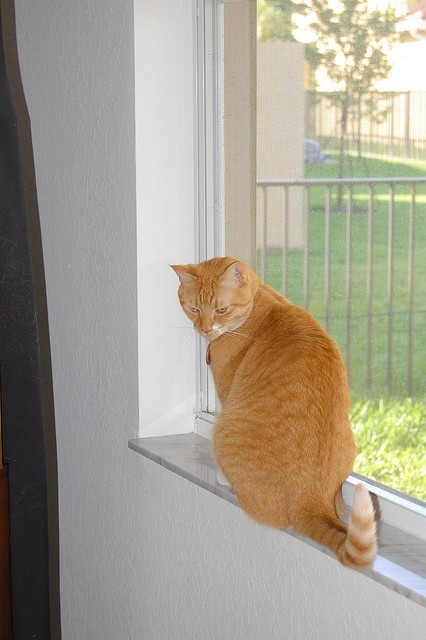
[168,256,381,572]
